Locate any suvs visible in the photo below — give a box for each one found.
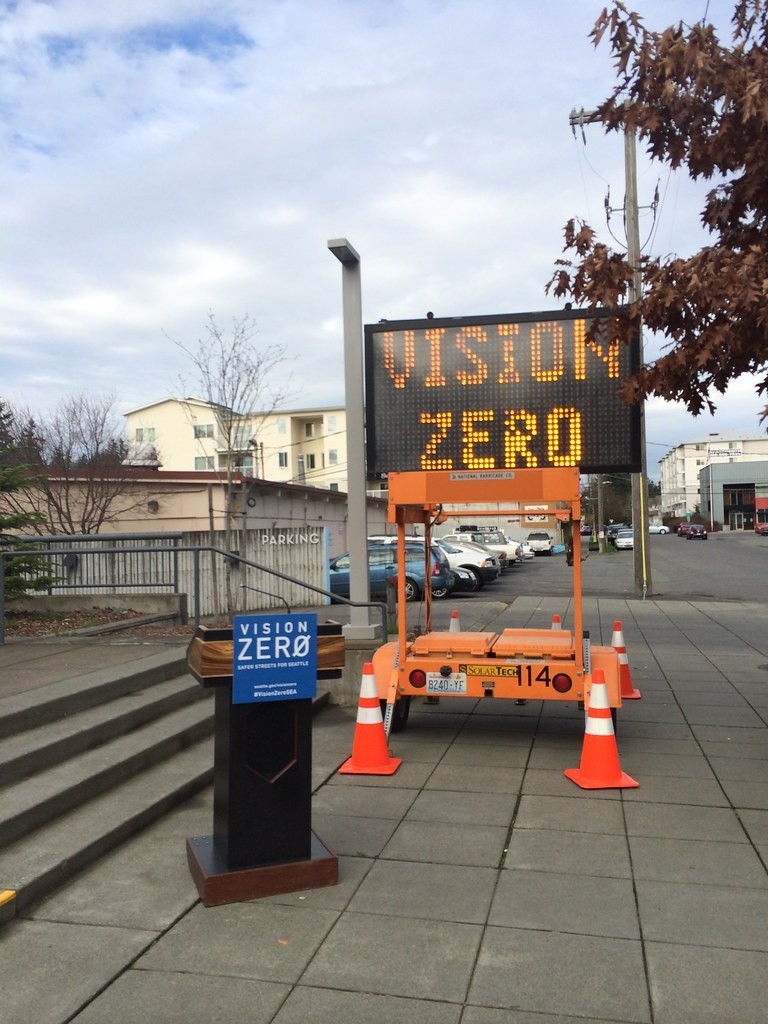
[442,528,554,575]
[365,533,499,589]
[328,544,455,602]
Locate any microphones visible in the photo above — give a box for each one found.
[239,583,290,614]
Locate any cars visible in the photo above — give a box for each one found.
[755,524,768,534]
[648,522,670,534]
[580,522,635,549]
[686,524,707,540]
[429,568,477,601]
[761,526,768,535]
[678,523,694,536]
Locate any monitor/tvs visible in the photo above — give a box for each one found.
[364,304,643,480]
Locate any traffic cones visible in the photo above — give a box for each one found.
[609,620,642,699]
[337,661,403,775]
[562,668,640,791]
[448,610,461,632]
[549,614,562,630]
